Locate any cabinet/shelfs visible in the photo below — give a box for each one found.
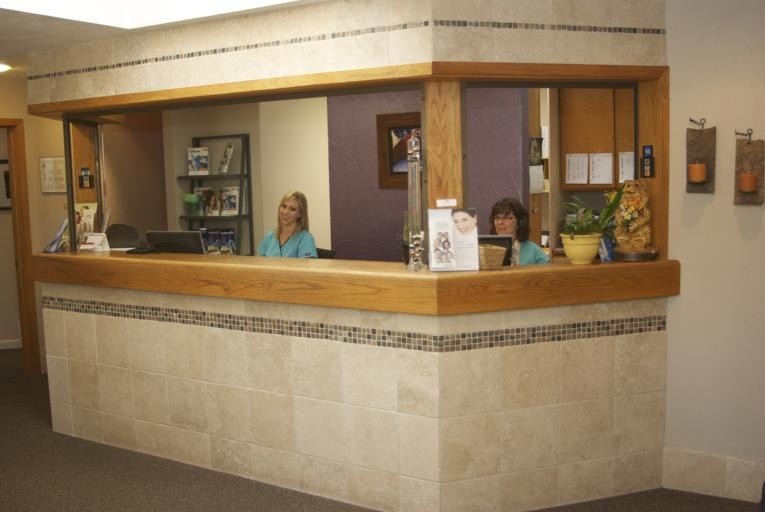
[177,133,255,255]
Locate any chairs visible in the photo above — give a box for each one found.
[105,224,139,248]
[316,247,335,259]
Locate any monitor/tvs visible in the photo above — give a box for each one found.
[478,235,512,266]
[147,231,206,254]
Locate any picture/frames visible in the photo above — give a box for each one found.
[376,112,422,190]
[0,159,11,209]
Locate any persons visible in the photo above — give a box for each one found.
[488,197,551,266]
[256,190,318,258]
[451,209,478,249]
[434,231,453,265]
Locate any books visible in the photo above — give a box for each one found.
[186,142,245,255]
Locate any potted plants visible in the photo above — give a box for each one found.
[558,189,629,265]
